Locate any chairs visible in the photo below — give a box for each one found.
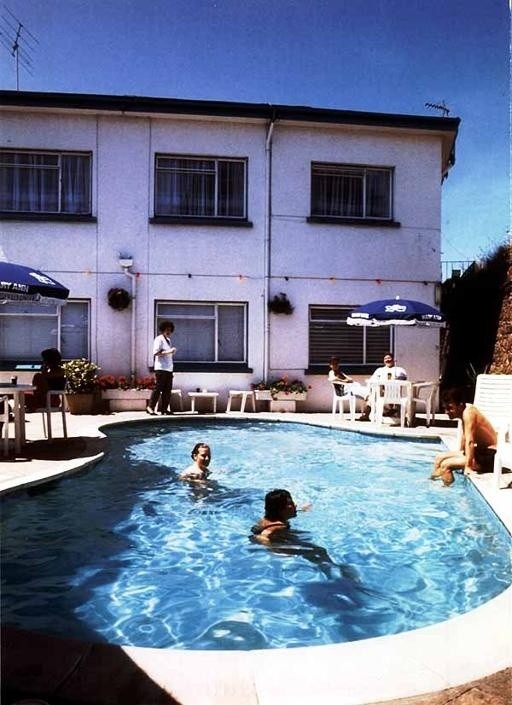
[0,372,67,461]
[374,378,414,428]
[494,425,512,494]
[452,370,512,450]
[412,381,441,427]
[331,381,372,422]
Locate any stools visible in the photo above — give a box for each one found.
[154,388,184,413]
[224,390,257,413]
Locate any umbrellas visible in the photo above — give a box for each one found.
[0,261,73,307]
[346,295,446,365]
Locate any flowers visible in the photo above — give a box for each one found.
[251,374,312,401]
[98,373,156,391]
[56,356,101,391]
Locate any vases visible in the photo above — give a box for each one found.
[65,390,95,415]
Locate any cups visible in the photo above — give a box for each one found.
[196,386,201,392]
[388,373,391,380]
[203,386,208,393]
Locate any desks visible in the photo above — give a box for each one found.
[365,378,424,425]
[188,391,219,413]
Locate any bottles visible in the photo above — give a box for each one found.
[10,376,17,385]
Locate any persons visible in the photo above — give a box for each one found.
[19,348,66,412]
[327,356,375,402]
[430,385,499,488]
[358,352,409,422]
[249,487,313,548]
[174,441,228,488]
[145,320,178,416]
